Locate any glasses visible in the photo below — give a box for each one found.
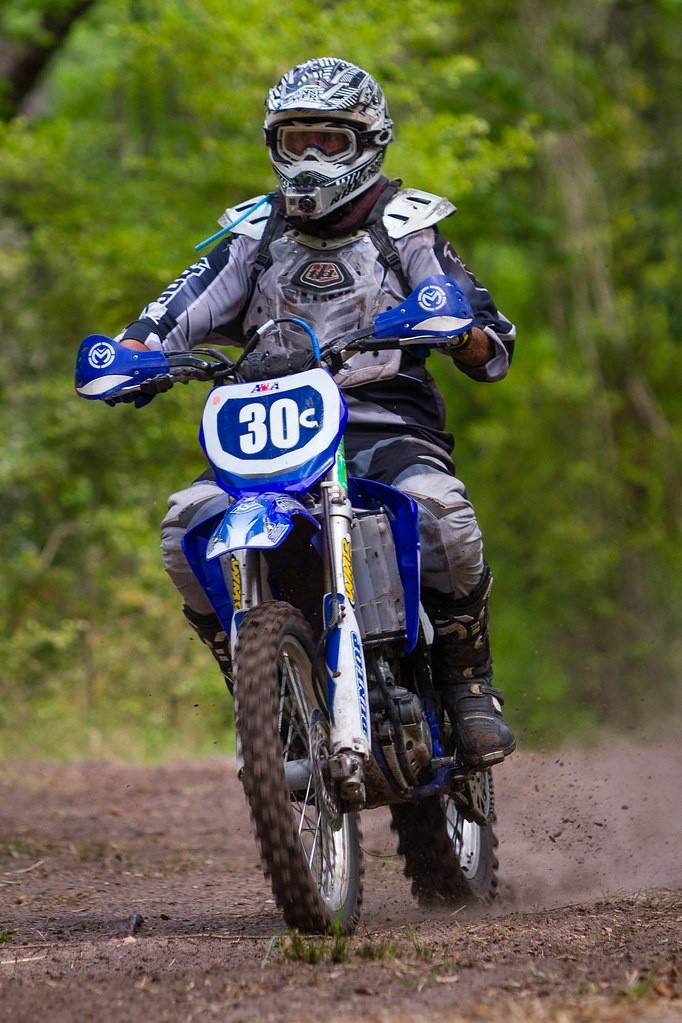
[275,124,365,166]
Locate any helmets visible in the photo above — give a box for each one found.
[264,57,394,218]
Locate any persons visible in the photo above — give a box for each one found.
[110,57,518,777]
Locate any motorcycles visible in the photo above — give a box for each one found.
[75,278,501,932]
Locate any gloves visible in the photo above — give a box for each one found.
[103,376,168,408]
[436,328,474,352]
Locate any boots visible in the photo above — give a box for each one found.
[419,559,518,767]
[184,604,233,698]
